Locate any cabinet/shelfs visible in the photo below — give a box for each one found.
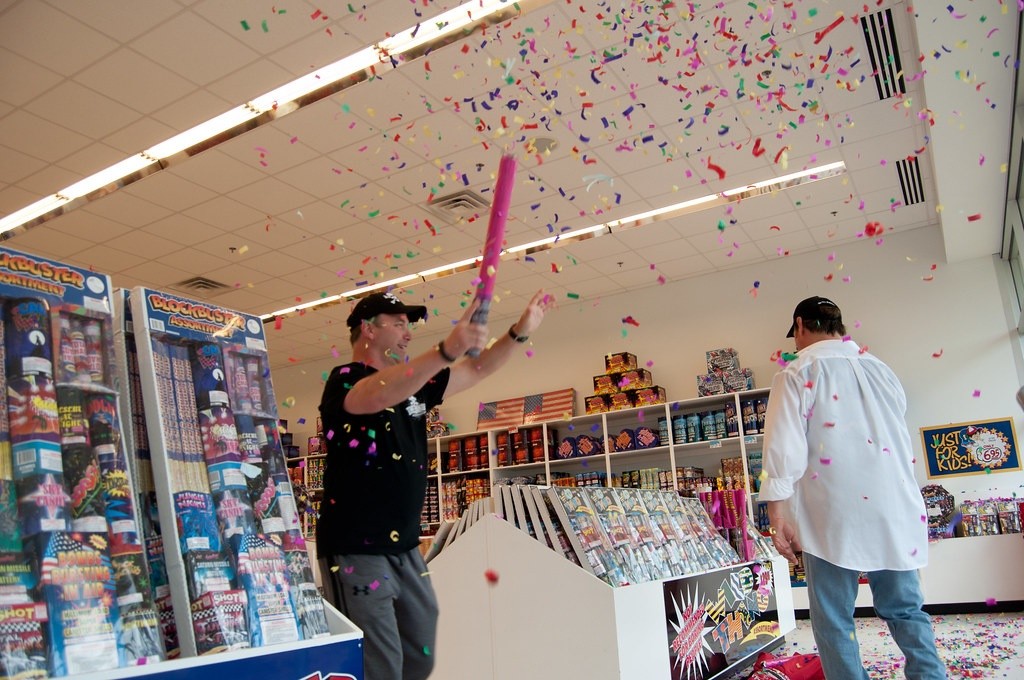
[289,386,872,587]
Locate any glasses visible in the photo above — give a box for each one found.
[373,322,417,335]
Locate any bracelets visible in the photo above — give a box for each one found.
[508,323,529,343]
[437,341,457,362]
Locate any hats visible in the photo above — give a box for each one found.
[786,296,838,338]
[347,293,426,334]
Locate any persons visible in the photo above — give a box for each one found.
[314,287,556,680]
[756,296,948,680]
